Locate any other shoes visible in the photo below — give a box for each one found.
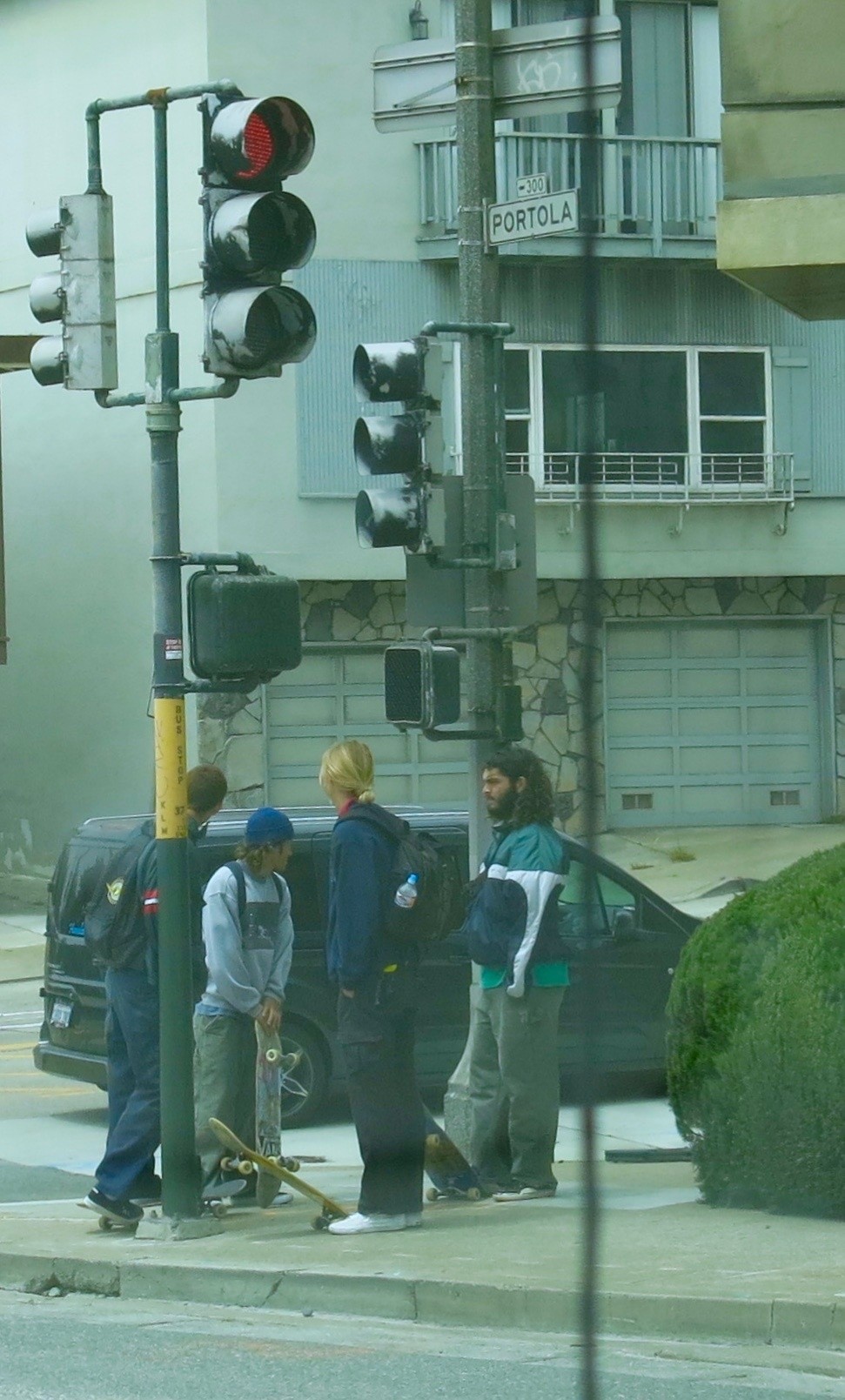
[407,1212,422,1227]
[228,1192,294,1207]
[328,1213,405,1236]
[492,1180,555,1200]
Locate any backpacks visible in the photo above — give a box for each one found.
[86,828,155,968]
[333,813,461,952]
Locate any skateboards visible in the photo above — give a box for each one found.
[422,1101,489,1200]
[254,1017,300,1209]
[75,1180,246,1230]
[209,1116,353,1231]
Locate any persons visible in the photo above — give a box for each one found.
[81,766,227,1223]
[316,741,429,1234]
[462,748,571,1202]
[188,805,296,1207]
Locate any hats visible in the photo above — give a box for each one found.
[244,808,294,847]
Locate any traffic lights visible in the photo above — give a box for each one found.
[348,334,430,559]
[20,191,123,394]
[199,93,321,385]
[384,644,463,734]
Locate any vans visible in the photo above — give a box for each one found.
[31,803,709,1140]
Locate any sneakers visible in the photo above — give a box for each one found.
[130,1174,161,1207]
[82,1185,143,1227]
